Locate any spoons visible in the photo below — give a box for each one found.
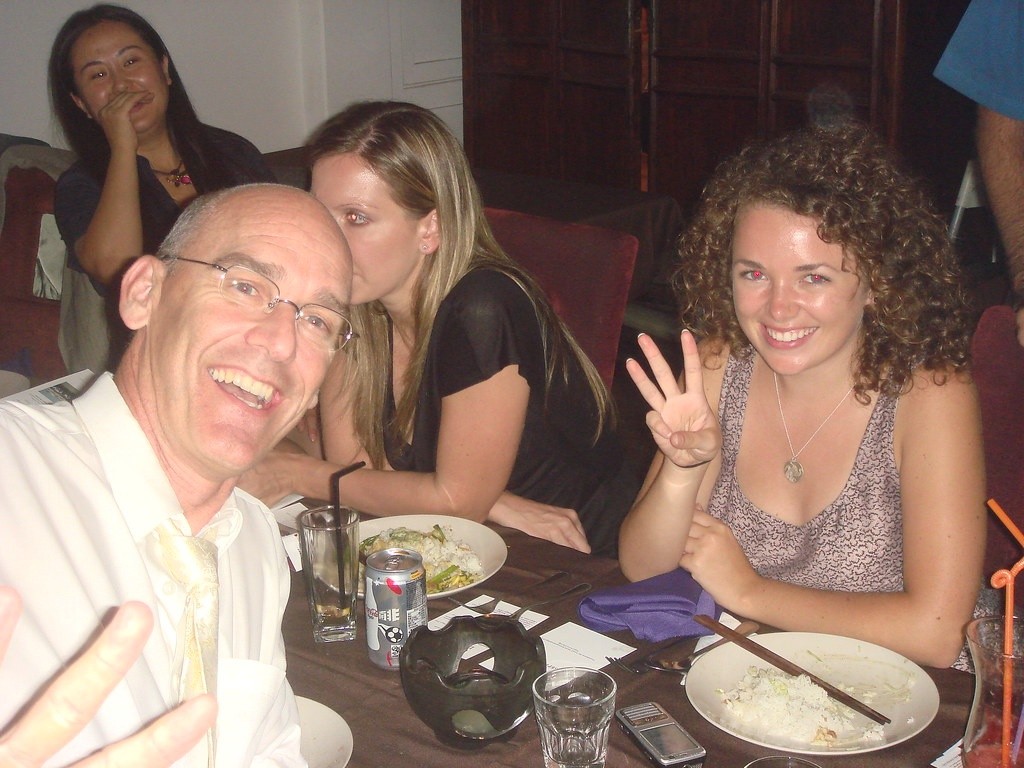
[484,581,593,619]
[644,620,761,672]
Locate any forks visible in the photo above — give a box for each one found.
[446,572,570,614]
[605,632,700,674]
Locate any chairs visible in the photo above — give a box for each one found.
[967,306,1024,615]
[0,129,315,387]
[482,205,637,390]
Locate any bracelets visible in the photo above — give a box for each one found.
[673,460,712,468]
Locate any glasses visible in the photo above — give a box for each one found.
[160,252,353,352]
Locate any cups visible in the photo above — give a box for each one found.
[743,756,821,768]
[532,666,617,768]
[299,504,360,642]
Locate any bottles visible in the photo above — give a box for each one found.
[962,615,1024,767]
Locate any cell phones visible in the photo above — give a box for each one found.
[616,702,706,768]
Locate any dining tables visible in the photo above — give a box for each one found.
[0,347,1024,767]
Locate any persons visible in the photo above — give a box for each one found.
[0,182,353,768]
[617,112,989,669]
[236,100,637,560]
[932,0,1024,348]
[47,3,326,463]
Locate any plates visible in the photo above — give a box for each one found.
[685,632,940,753]
[318,514,507,599]
[0,369,31,399]
[293,694,354,768]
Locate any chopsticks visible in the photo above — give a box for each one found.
[693,613,892,724]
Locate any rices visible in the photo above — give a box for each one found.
[715,672,914,747]
[378,526,482,575]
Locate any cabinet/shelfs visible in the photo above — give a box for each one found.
[460,0,906,327]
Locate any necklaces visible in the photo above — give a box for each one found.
[773,370,859,484]
[151,158,192,187]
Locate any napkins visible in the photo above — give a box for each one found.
[3,348,34,381]
[578,565,723,642]
[681,612,757,684]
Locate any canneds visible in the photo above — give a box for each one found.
[364,547,427,671]
